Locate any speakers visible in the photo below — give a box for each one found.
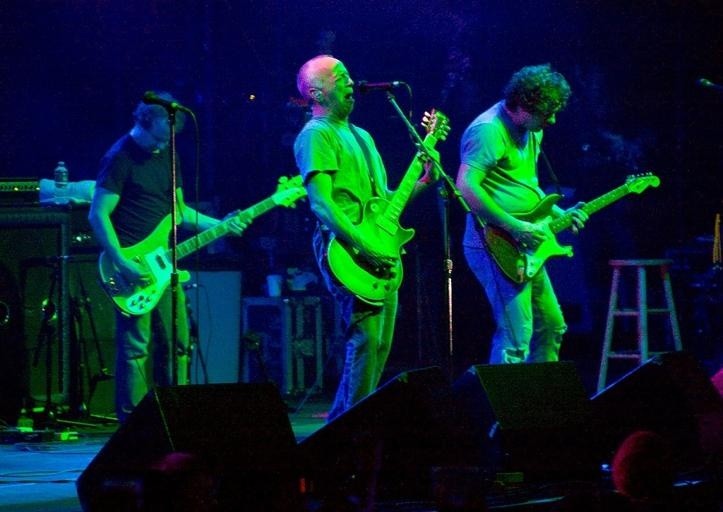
[591,351,723,483]
[76,382,302,512]
[453,361,596,472]
[298,367,497,498]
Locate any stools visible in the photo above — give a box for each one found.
[594,257,683,392]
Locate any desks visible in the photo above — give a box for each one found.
[241,295,324,396]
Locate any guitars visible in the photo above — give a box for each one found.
[480,171,661,288]
[98,174,310,318]
[322,110,451,310]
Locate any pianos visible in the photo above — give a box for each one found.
[0,203,217,226]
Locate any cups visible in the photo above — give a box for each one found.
[265,275,284,299]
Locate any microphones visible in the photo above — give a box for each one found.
[143,91,192,114]
[358,80,399,94]
[694,78,723,97]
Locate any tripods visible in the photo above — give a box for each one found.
[385,90,471,374]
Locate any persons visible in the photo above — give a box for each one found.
[294,53,442,422]
[456,62,589,366]
[89,87,252,428]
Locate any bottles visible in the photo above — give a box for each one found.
[53,161,70,206]
